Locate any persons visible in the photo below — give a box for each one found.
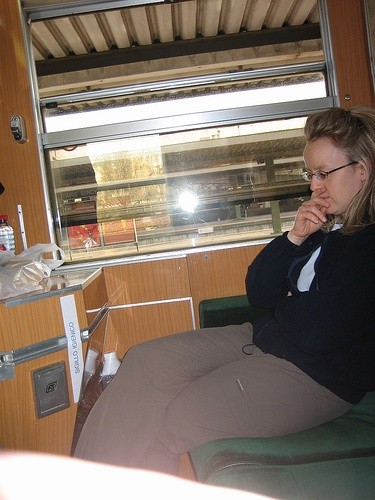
[69,107,375,500]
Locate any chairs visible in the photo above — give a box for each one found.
[189,292,375,500]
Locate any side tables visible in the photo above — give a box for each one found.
[0,266,110,458]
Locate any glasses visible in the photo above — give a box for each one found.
[301,162,358,181]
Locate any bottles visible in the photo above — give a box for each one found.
[0,216,15,255]
[100,351,121,391]
[80,347,99,399]
